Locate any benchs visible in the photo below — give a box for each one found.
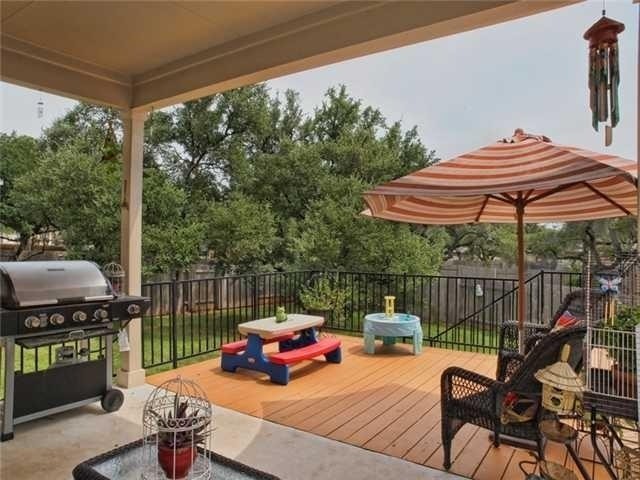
[221,332,295,374]
[268,338,343,385]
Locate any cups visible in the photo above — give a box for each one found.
[276,306,286,321]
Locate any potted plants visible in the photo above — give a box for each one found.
[147,394,215,479]
[594,300,640,398]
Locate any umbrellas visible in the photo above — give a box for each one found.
[355,128,639,358]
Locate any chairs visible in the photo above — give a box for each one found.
[438,326,586,469]
[499,288,615,365]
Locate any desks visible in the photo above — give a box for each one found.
[238,313,324,369]
[363,312,423,356]
